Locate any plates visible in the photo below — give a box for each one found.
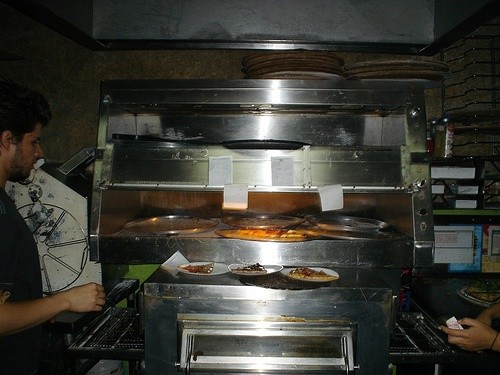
[177,262,230,275]
[280,268,339,283]
[228,264,283,276]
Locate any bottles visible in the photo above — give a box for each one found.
[431,117,455,158]
[426,118,437,154]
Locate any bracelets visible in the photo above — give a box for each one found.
[491,331,499,350]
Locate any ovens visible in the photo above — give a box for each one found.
[66,78,462,375]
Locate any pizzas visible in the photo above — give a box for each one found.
[182,262,214,274]
[289,268,337,282]
[223,229,317,238]
[466,286,500,301]
[232,263,267,276]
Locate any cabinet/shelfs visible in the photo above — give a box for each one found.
[87,76,437,273]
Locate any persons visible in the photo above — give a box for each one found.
[0,78,107,373]
[436,302,500,354]
